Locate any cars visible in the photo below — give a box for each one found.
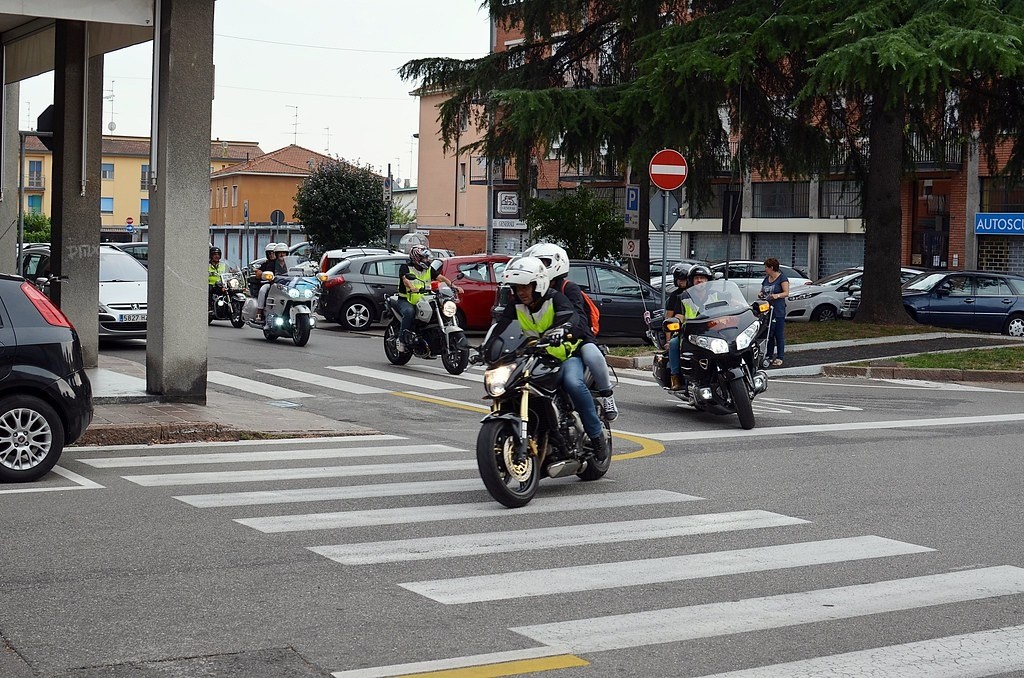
[110,240,149,269]
[618,257,815,310]
[837,270,1024,338]
[559,258,670,347]
[428,254,515,335]
[313,246,454,331]
[245,240,321,296]
[0,272,93,485]
[781,265,929,322]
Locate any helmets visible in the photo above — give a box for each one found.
[265,242,277,254]
[209,247,222,260]
[409,244,433,270]
[274,243,289,254]
[690,265,714,281]
[521,243,570,283]
[501,255,550,300]
[670,262,692,286]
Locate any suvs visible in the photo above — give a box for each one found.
[15,241,153,344]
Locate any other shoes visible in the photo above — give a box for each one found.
[772,359,783,366]
[255,313,263,321]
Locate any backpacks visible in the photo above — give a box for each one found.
[562,278,600,337]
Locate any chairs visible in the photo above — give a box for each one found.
[469,265,490,281]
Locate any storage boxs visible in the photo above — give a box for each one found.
[241,296,258,322]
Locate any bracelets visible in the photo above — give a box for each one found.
[778,294,780,298]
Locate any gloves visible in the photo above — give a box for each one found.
[541,326,565,348]
[467,354,484,365]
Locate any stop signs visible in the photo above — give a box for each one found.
[126,217,133,225]
[650,150,688,190]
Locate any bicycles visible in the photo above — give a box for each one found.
[28,274,71,301]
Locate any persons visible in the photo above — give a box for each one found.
[663,263,754,391]
[396,245,464,352]
[255,242,290,330]
[208,247,242,314]
[758,258,789,365]
[467,243,618,460]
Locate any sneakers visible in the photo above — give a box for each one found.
[599,390,619,422]
[590,432,608,461]
[395,338,405,353]
[671,374,682,391]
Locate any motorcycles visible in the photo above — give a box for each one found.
[239,256,323,347]
[642,278,774,430]
[381,273,471,375]
[207,264,247,329]
[463,309,613,507]
